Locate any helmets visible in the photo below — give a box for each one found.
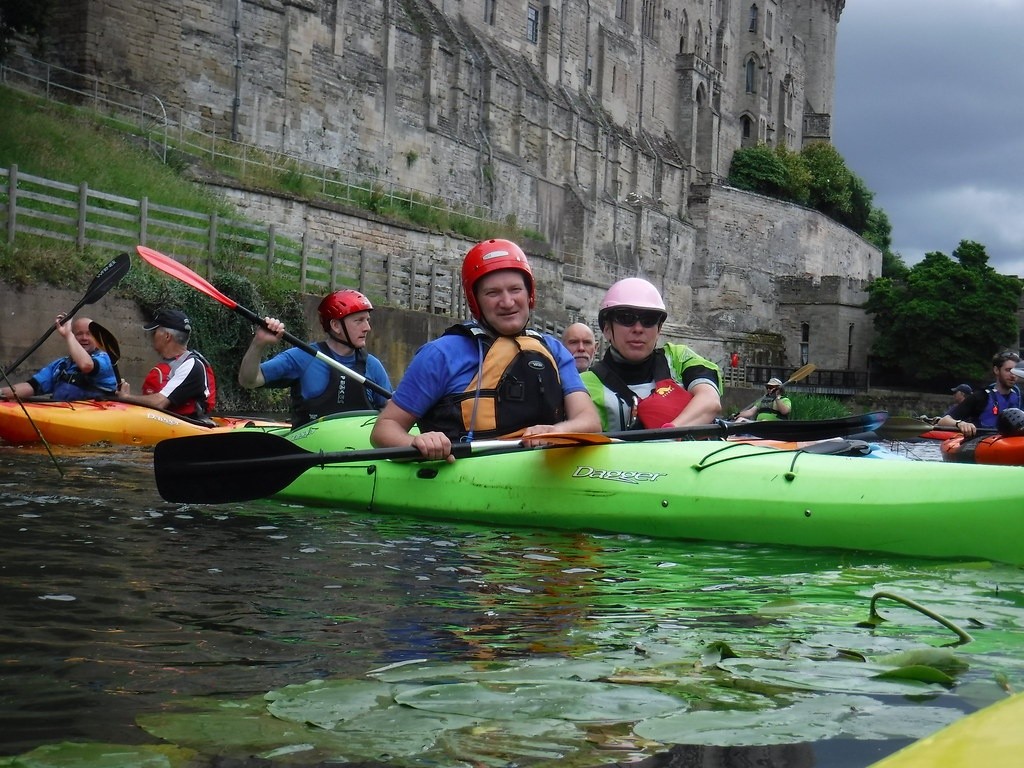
[461,239,535,320]
[598,277,667,332]
[317,290,374,333]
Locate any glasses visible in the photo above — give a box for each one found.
[607,311,661,328]
[766,386,776,390]
[999,352,1019,357]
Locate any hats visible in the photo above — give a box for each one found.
[766,378,783,386]
[951,384,973,395]
[142,310,191,332]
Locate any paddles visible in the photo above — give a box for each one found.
[913,416,940,422]
[134,244,396,401]
[0,251,132,384]
[152,409,893,505]
[1010,360,1024,379]
[88,321,124,392]
[522,430,623,444]
[727,364,816,421]
[873,415,999,441]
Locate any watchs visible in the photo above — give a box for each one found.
[956,420,962,428]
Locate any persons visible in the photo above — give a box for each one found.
[238,289,393,433]
[578,277,722,441]
[731,378,791,425]
[559,323,599,374]
[939,349,1024,436]
[115,308,216,425]
[370,239,602,463]
[0,313,119,404]
[943,384,977,419]
